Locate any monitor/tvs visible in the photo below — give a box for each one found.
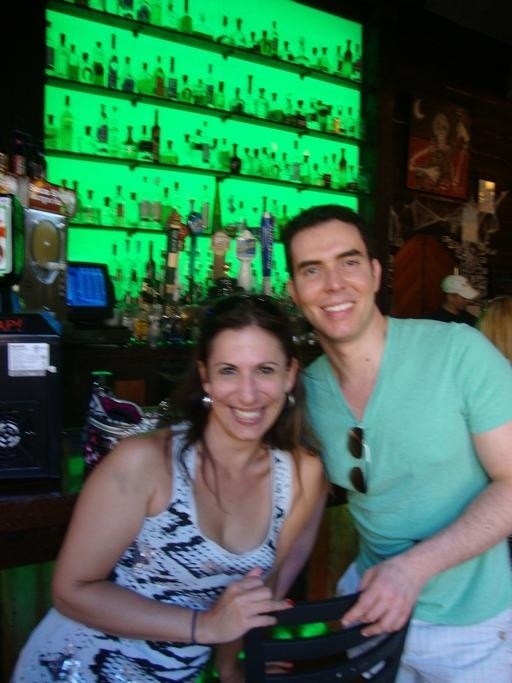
[63,261,118,318]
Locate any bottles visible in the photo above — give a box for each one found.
[10,138,27,177]
[0,151,28,287]
[45,0,364,345]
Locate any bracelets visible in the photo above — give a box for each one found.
[189,607,199,646]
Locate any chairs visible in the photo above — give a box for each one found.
[244,592,413,682]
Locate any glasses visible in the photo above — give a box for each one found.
[347,425,373,495]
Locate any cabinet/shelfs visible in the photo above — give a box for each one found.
[2,0,399,331]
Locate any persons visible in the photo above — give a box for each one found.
[9,291,328,682]
[432,271,481,326]
[277,202,512,682]
